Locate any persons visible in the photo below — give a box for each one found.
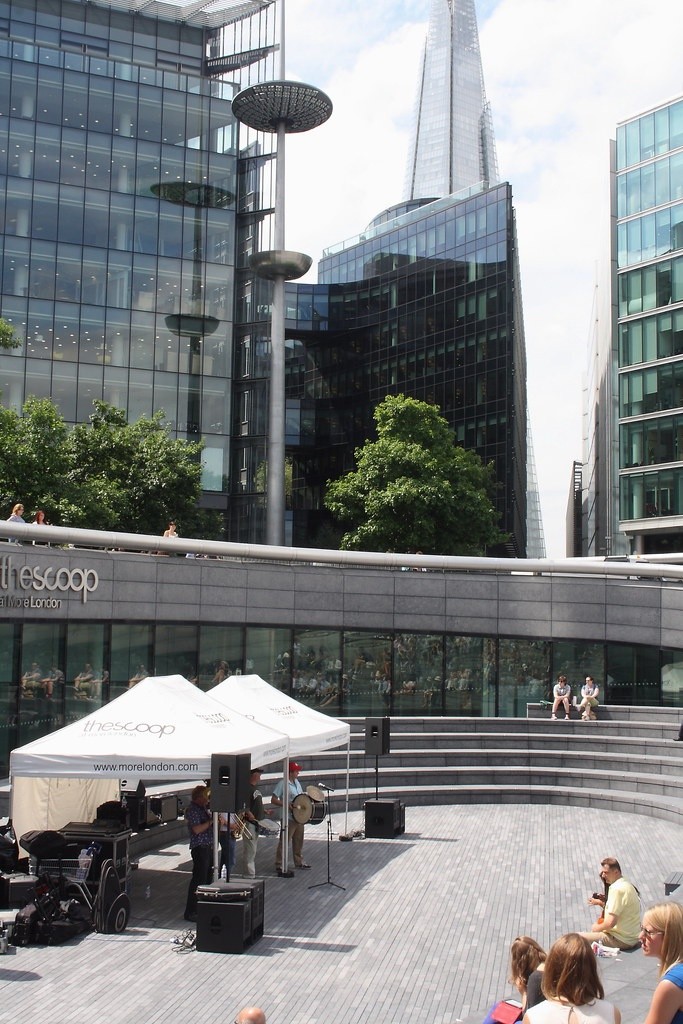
[481,857,683,1024]
[155,522,208,559]
[674,720,683,741]
[31,511,49,546]
[18,634,614,721]
[6,504,28,543]
[235,1008,267,1024]
[189,763,310,922]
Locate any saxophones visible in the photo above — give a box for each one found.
[231,813,246,838]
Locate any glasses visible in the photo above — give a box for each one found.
[640,926,665,935]
[558,681,565,683]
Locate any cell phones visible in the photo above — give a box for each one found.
[504,999,523,1008]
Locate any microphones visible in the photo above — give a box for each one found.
[318,783,334,791]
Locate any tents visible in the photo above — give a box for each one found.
[10,675,350,884]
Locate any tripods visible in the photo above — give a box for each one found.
[309,790,346,890]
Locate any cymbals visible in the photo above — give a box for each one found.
[306,785,326,802]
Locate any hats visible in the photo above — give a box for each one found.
[434,676,441,681]
[251,768,264,774]
[289,762,302,771]
[342,675,348,679]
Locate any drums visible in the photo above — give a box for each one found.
[291,793,327,825]
[257,819,280,838]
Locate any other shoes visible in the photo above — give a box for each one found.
[565,714,569,719]
[296,864,312,870]
[242,874,255,879]
[575,705,581,711]
[277,867,294,873]
[585,716,590,721]
[184,912,197,921]
[551,716,557,720]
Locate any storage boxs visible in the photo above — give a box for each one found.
[29,836,128,895]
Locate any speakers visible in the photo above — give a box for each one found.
[364,798,405,839]
[365,717,389,754]
[120,777,145,798]
[210,754,250,811]
[194,879,265,955]
[119,797,147,832]
[149,794,177,822]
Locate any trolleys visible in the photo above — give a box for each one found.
[28,840,104,912]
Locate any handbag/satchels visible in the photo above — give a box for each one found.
[19,829,64,858]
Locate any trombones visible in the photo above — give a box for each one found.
[230,812,253,841]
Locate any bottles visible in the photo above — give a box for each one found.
[221,864,227,881]
[144,885,151,900]
[597,940,604,958]
[573,696,577,706]
